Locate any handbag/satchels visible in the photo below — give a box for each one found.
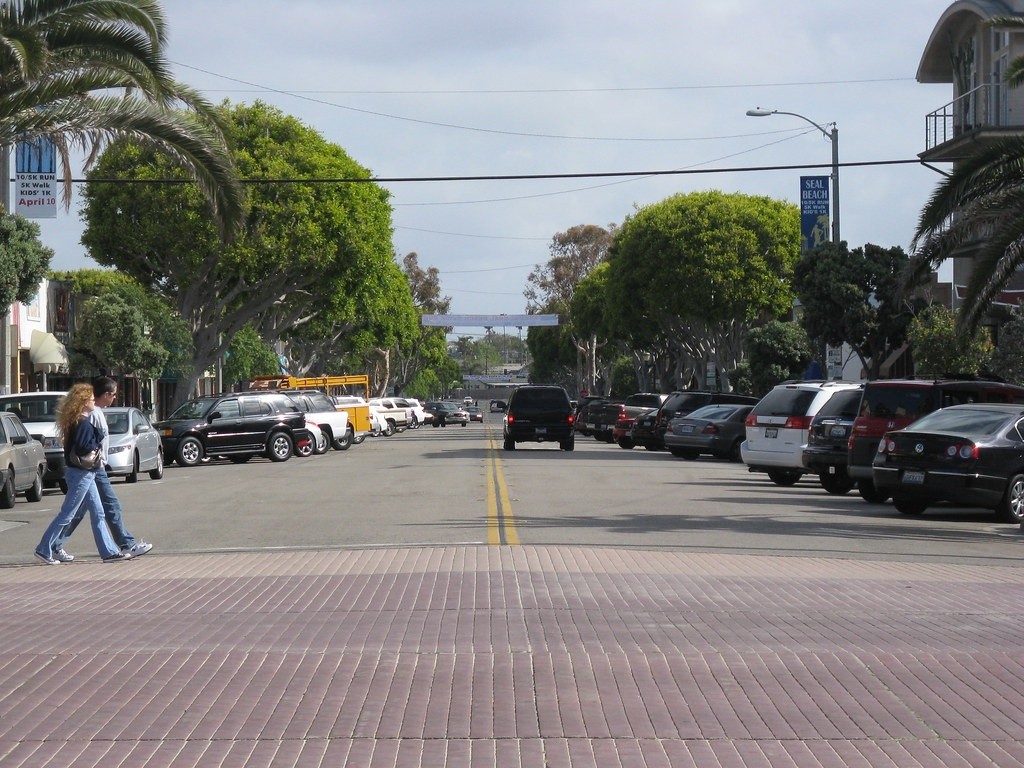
[70,445,102,472]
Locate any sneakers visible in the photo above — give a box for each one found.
[122,543,152,556]
[34,551,61,565]
[103,553,131,562]
[52,549,74,562]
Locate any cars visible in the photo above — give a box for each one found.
[663,404,756,463]
[570,390,670,450]
[462,406,484,424]
[872,403,1024,524]
[0,411,47,510]
[452,396,507,413]
[95,406,166,483]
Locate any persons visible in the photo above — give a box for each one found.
[34,375,154,565]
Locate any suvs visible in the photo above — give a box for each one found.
[248,374,434,455]
[422,400,471,428]
[847,381,1024,508]
[149,391,309,468]
[803,389,889,504]
[0,391,86,493]
[740,378,867,487]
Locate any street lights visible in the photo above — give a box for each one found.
[746,107,840,246]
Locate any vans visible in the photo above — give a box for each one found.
[497,385,578,452]
[653,390,763,459]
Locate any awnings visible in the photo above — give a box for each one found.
[29,329,70,374]
[861,340,910,379]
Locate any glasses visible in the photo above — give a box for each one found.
[108,393,116,396]
